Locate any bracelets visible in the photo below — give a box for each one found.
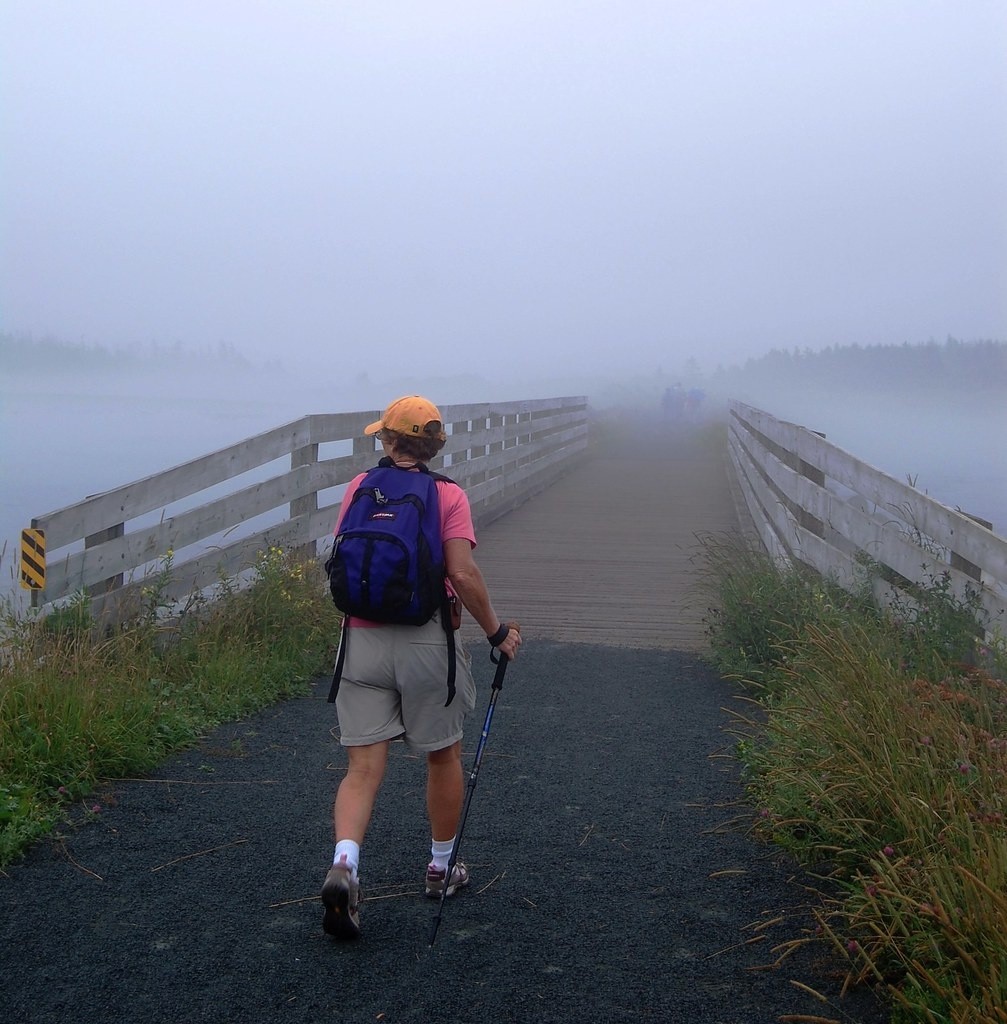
[486,623,510,647]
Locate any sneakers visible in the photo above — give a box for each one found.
[321,851,361,939]
[425,862,469,898]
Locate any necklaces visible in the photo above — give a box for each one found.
[395,461,420,472]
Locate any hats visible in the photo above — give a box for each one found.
[364,395,447,442]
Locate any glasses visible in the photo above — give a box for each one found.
[374,428,394,440]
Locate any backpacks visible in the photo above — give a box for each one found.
[326,456,442,627]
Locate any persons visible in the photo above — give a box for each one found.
[322,394,521,939]
